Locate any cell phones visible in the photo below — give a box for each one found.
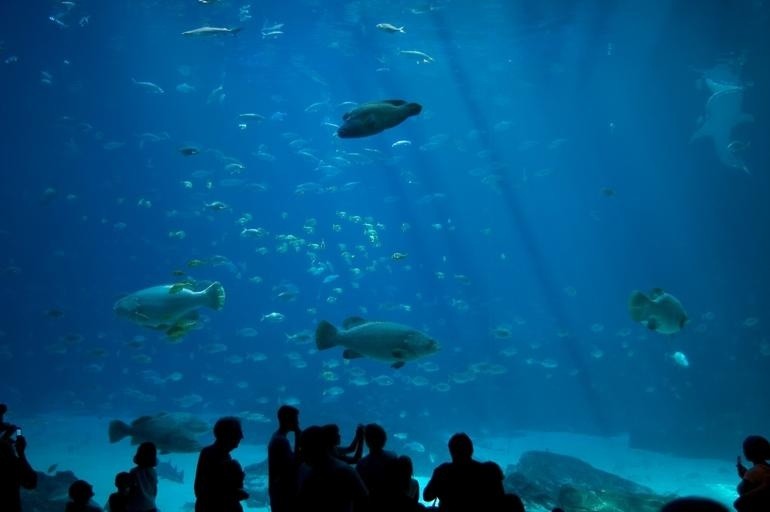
[16,427,22,438]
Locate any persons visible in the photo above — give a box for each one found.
[129,441,158,512]
[191,404,526,512]
[109,472,129,512]
[66,479,100,512]
[0,435,38,512]
[732,434,770,512]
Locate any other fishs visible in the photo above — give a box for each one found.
[31,0,716,465]
